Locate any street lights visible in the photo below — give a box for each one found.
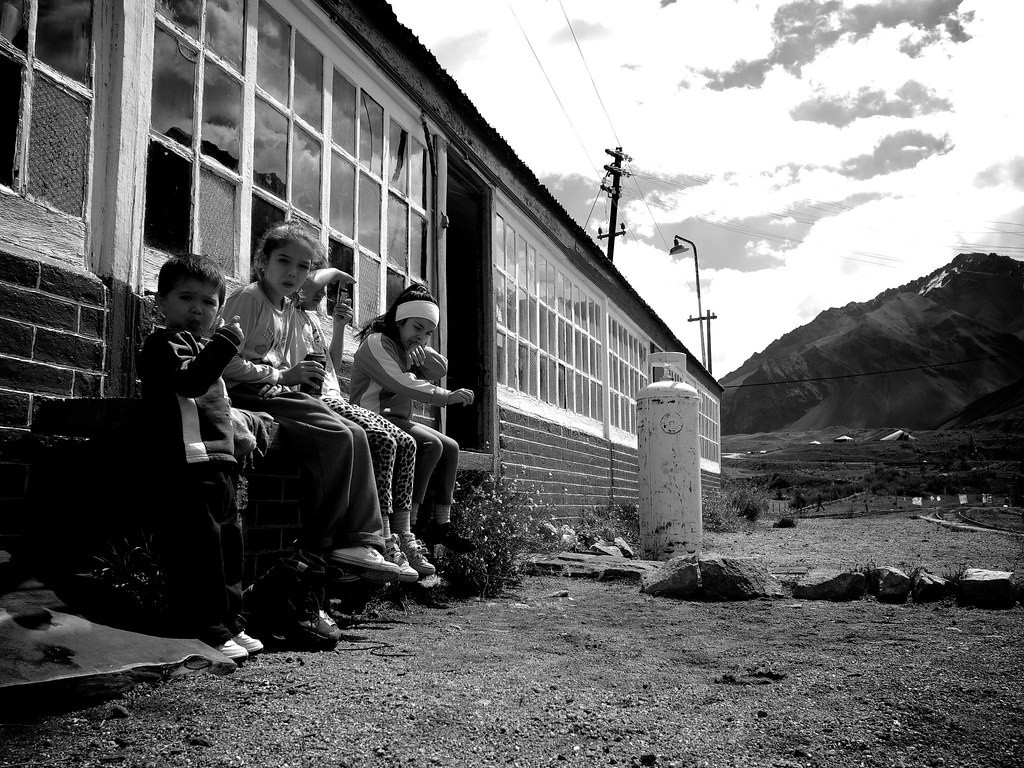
[668,234,708,369]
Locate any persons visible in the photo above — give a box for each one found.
[138,252,264,660]
[287,246,436,583]
[215,220,400,581]
[349,283,478,568]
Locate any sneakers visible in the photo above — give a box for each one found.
[232,631,264,656]
[213,638,249,663]
[328,541,400,582]
[382,533,419,582]
[392,531,435,575]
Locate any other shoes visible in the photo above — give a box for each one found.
[430,518,477,551]
[411,522,435,547]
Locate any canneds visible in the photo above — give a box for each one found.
[300,354,326,396]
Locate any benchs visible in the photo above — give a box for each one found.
[34,396,496,594]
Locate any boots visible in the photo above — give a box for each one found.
[276,551,340,652]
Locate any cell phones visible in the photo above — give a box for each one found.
[336,289,348,318]
